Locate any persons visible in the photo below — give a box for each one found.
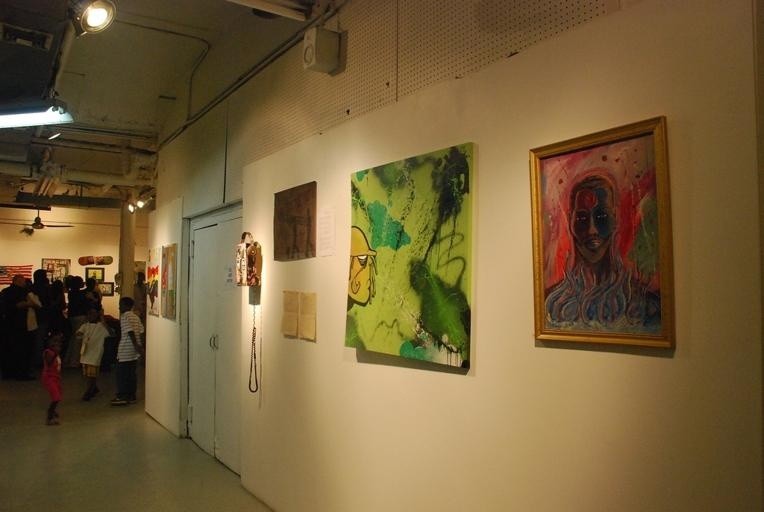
[0,269,102,381]
[543,174,658,302]
[39,333,65,426]
[71,307,117,401]
[110,296,145,406]
[133,272,146,359]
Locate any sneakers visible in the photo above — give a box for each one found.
[111,396,128,405]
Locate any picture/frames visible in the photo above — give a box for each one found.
[86,267,116,298]
[525,116,683,357]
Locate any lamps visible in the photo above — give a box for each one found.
[66,1,119,38]
